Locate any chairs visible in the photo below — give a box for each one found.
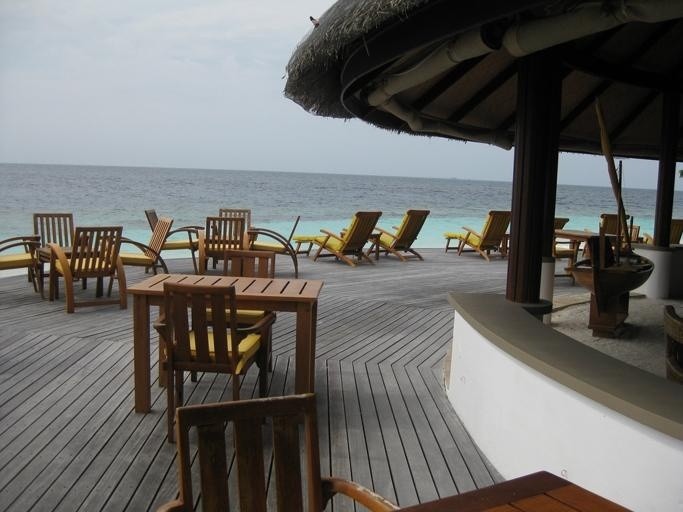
[151,280,278,443]
[142,206,303,278]
[369,204,436,263]
[311,208,383,267]
[105,215,174,297]
[0,234,45,301]
[32,210,76,284]
[152,392,402,512]
[48,225,129,315]
[456,209,513,262]
[188,249,277,383]
[553,213,646,283]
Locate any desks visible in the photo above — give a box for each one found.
[35,246,112,301]
[392,468,636,512]
[124,270,325,421]
